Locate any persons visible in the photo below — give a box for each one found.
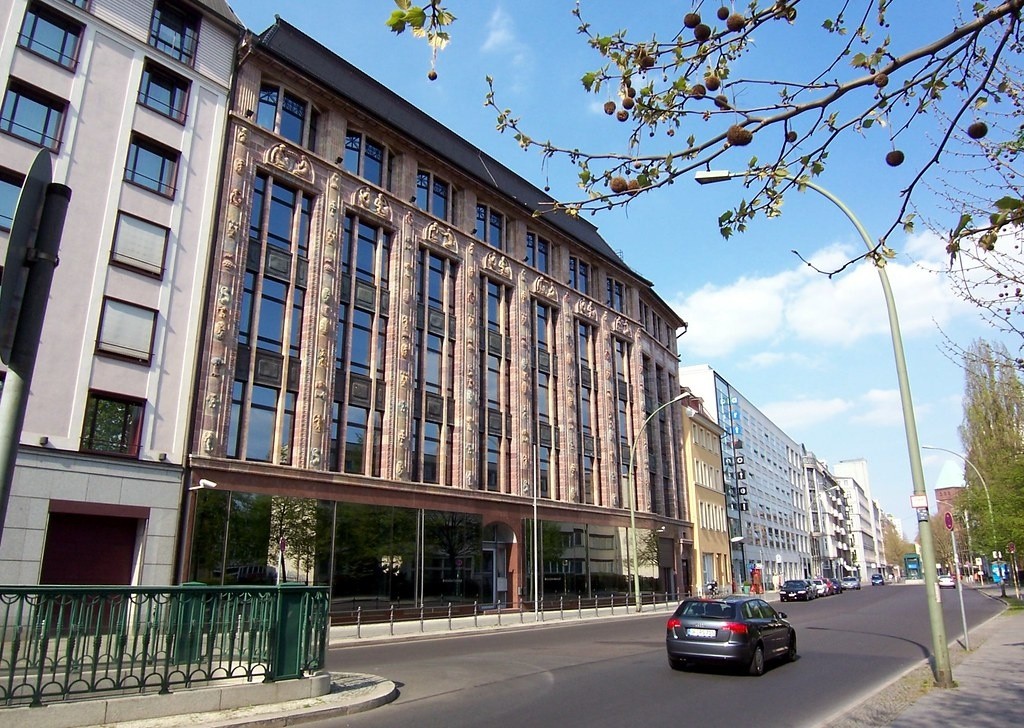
[807,571,918,580]
[773,573,779,594]
[708,580,717,599]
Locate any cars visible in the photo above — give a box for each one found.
[870,574,885,586]
[664,595,800,676]
[939,574,956,588]
[778,575,862,602]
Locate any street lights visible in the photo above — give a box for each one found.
[922,446,1007,597]
[932,498,976,584]
[693,168,955,687]
[627,390,691,612]
[805,486,841,579]
